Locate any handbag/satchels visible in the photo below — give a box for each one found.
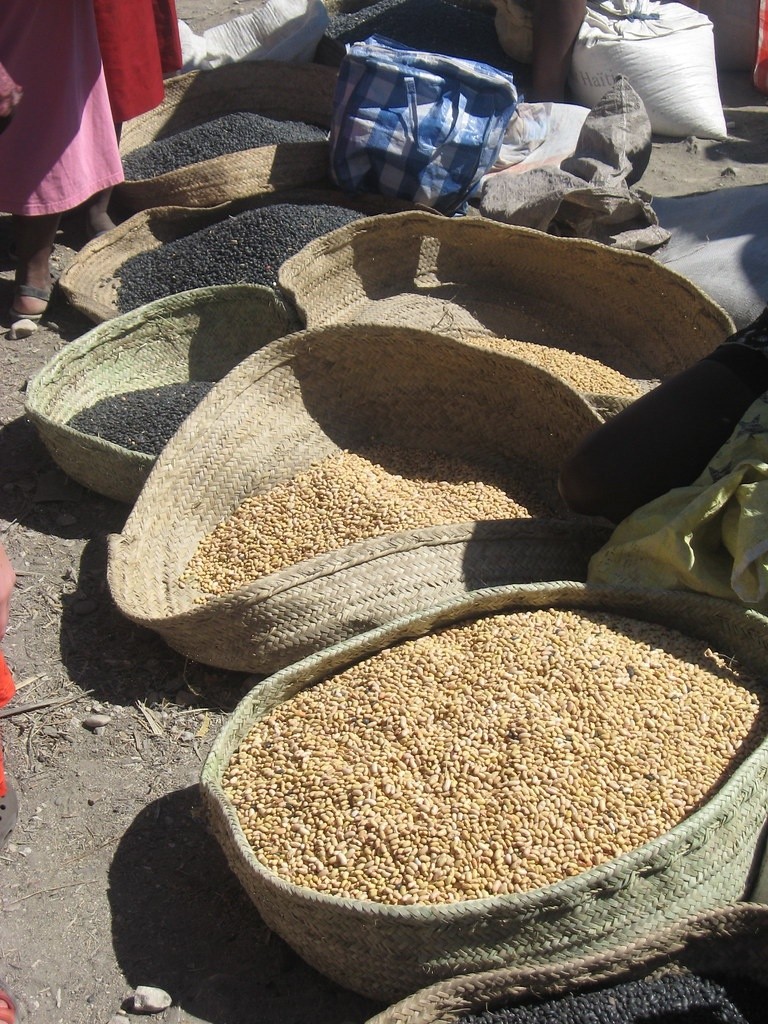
[331,33,519,218]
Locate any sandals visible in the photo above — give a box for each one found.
[8,270,58,329]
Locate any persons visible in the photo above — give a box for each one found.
[0,0,183,319]
[492,0,586,103]
